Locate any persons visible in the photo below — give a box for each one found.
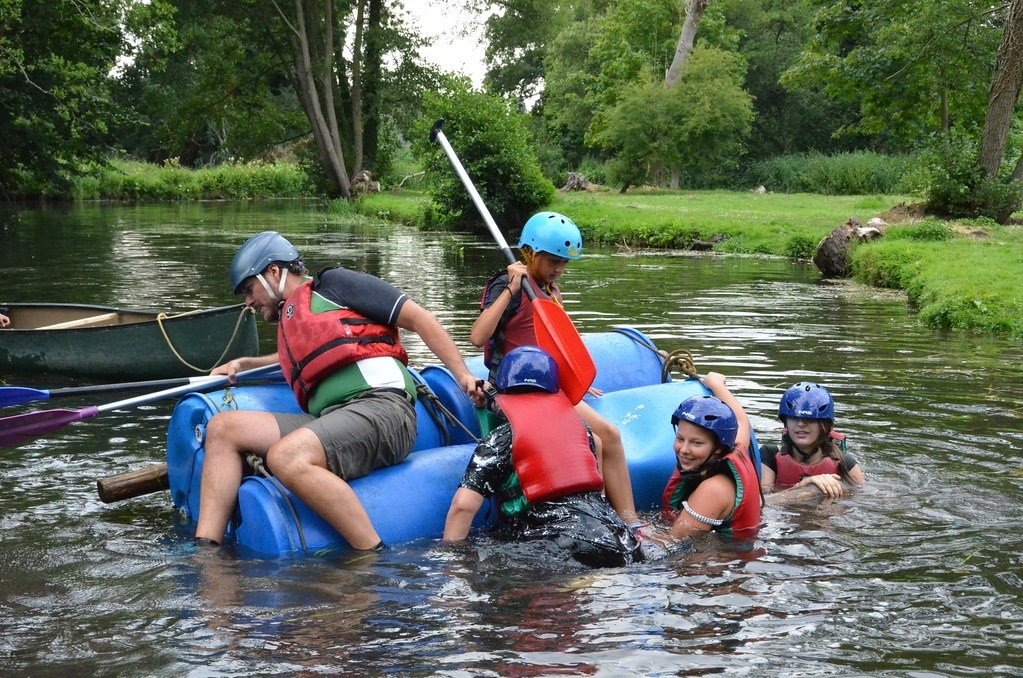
[196,231,495,550]
[443,212,637,542]
[758,381,865,497]
[663,372,760,532]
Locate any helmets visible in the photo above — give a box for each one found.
[494,343,559,391]
[672,394,741,448]
[516,211,583,259]
[780,382,836,419]
[229,231,299,296]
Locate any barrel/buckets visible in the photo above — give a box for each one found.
[225,442,497,562]
[584,380,762,515]
[418,327,672,446]
[166,366,449,524]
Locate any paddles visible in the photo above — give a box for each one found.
[427,116,598,408]
[1,362,287,450]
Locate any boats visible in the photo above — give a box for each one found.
[0,298,261,389]
